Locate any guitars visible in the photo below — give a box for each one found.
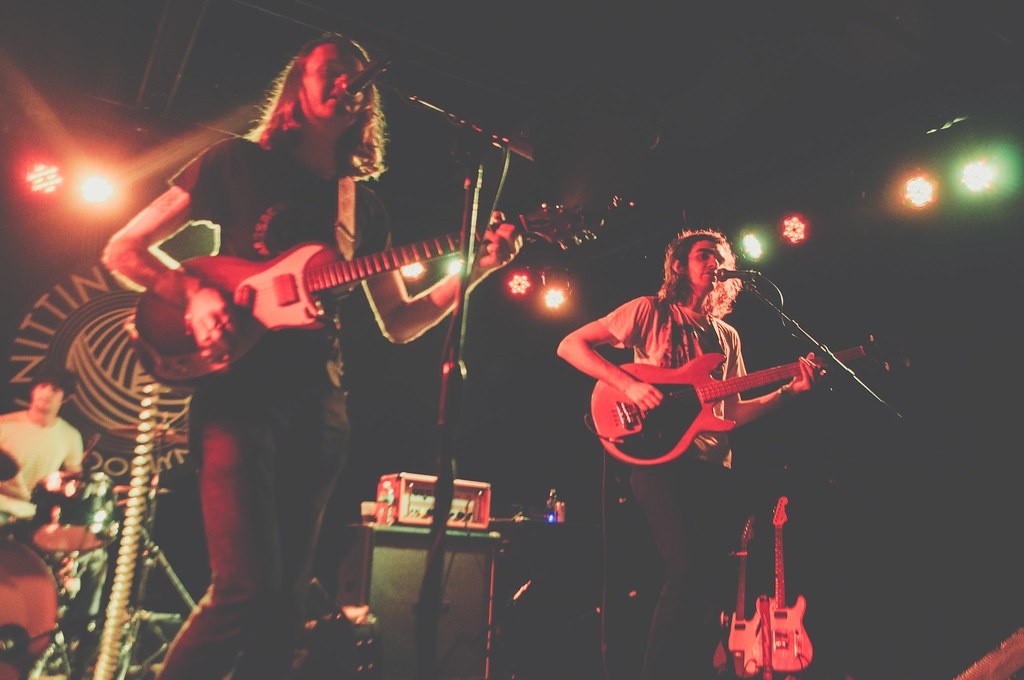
[752,494,817,673]
[589,330,914,468]
[708,507,763,677]
[135,194,629,390]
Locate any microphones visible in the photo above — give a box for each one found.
[716,268,761,282]
[346,59,388,97]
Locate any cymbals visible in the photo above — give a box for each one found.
[0,442,21,484]
[108,423,188,445]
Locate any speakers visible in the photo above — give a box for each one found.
[336,520,500,680]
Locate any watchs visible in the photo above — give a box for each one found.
[777,386,797,403]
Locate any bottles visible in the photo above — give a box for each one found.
[544,489,557,523]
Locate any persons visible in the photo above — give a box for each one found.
[96,32,525,679]
[1,363,88,530]
[558,228,827,680]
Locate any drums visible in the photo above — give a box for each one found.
[28,472,119,551]
[0,534,63,680]
